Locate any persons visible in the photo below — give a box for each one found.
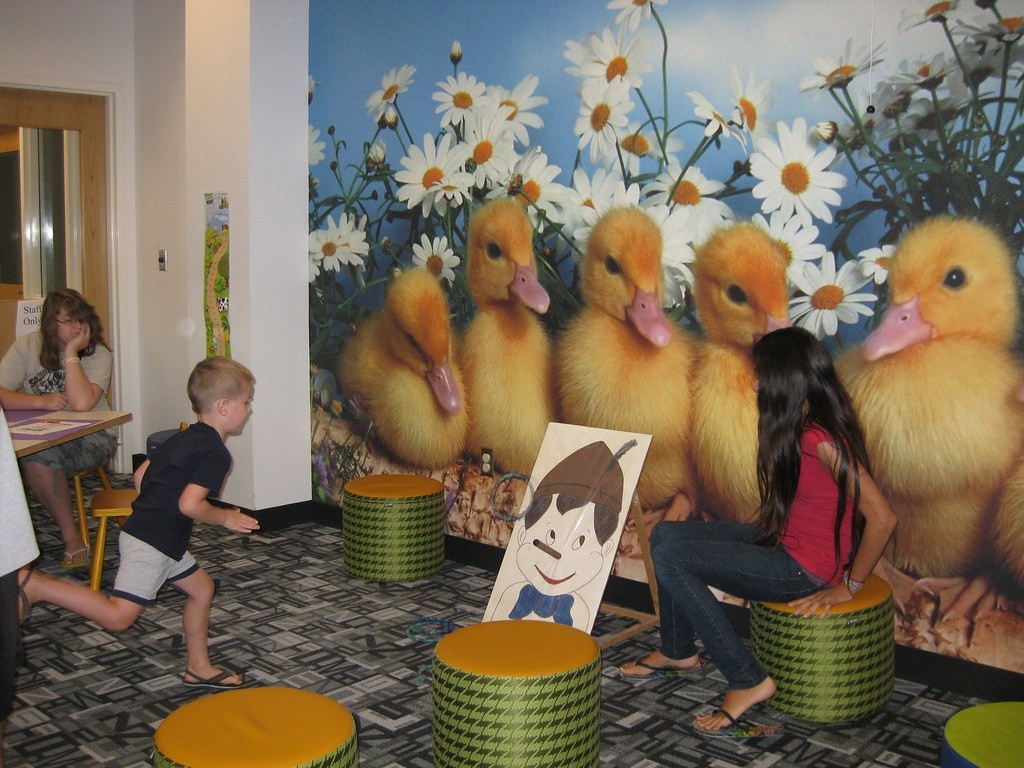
[17,357,260,689]
[0,288,119,569]
[618,325,899,737]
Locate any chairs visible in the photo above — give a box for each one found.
[87,421,189,591]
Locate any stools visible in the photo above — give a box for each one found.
[746,572,894,723]
[939,701,1024,768]
[341,474,446,583]
[429,620,602,768]
[152,685,359,768]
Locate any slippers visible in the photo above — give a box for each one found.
[182,667,259,689]
[693,709,786,739]
[61,544,92,569]
[18,568,33,627]
[619,654,704,680]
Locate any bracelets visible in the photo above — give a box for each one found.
[843,571,864,593]
[62,357,80,365]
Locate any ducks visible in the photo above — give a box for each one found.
[335,194,1024,611]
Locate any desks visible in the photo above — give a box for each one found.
[4,408,136,461]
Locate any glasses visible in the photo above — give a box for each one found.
[55,317,85,326]
